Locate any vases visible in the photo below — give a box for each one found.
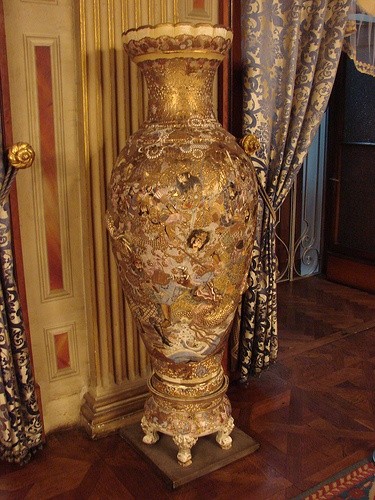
[101,20,261,469]
